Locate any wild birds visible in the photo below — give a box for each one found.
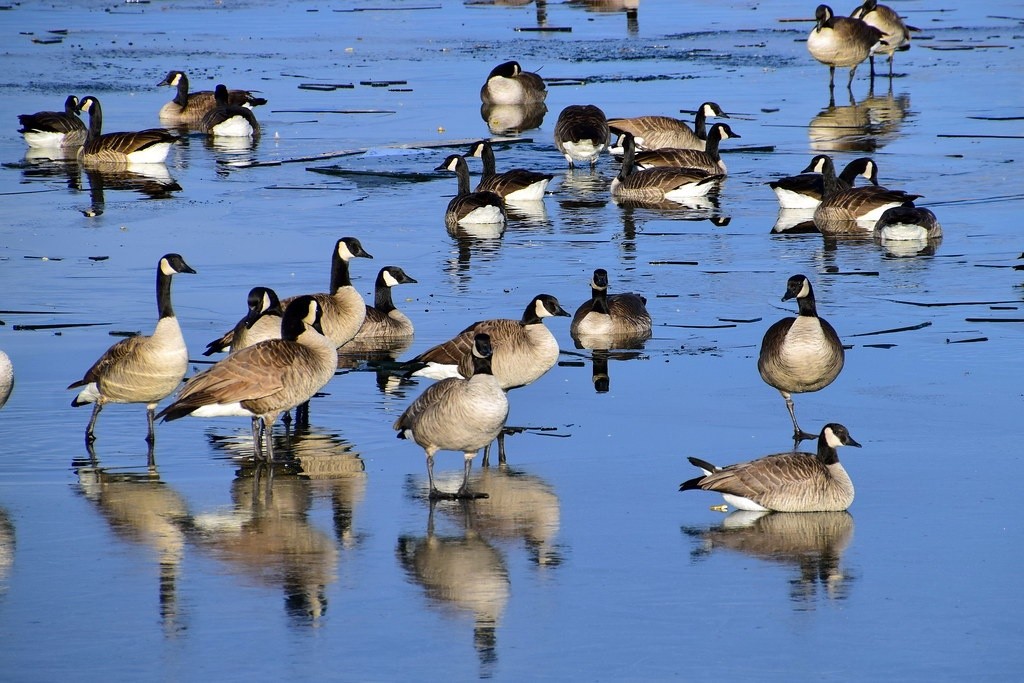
[756,274,844,438]
[434,154,506,224]
[681,511,854,600]
[807,0,920,88]
[66,251,338,467]
[394,333,509,501]
[157,71,268,137]
[480,61,548,104]
[481,102,548,136]
[677,423,862,511]
[604,102,742,201]
[570,268,652,350]
[462,141,554,200]
[15,95,87,146]
[68,456,561,679]
[202,237,417,357]
[554,105,610,175]
[397,292,572,468]
[73,96,180,163]
[763,154,942,239]
